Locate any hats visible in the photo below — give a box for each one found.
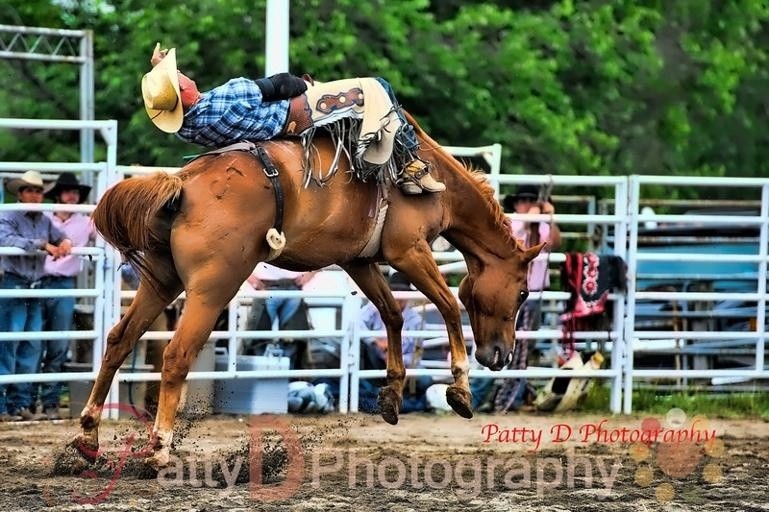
[44,172,91,203]
[388,271,410,290]
[4,170,56,196]
[140,46,184,133]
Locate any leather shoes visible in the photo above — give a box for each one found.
[403,159,446,193]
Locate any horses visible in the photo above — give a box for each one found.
[70,107,547,481]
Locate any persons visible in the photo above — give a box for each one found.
[41,171,98,418]
[121,183,720,413]
[140,39,448,199]
[0,169,73,423]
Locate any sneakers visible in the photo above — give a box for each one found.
[503,187,553,211]
[21,409,47,421]
[46,408,62,420]
[1,414,23,422]
